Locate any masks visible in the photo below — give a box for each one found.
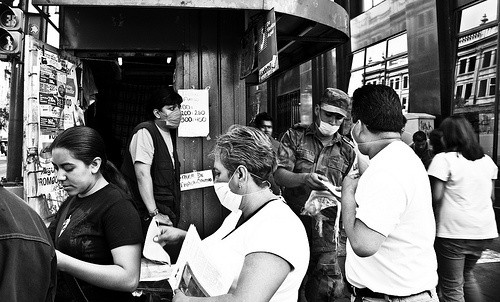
[213,167,243,212]
[160,108,182,128]
[350,123,358,152]
[318,111,340,136]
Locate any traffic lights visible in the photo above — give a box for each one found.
[0,1,26,61]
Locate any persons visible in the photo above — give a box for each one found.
[47,126,144,302]
[336,84,442,302]
[254,112,281,197]
[1,184,57,302]
[154,124,311,302]
[408,131,433,171]
[121,89,183,265]
[272,88,356,302]
[427,111,499,302]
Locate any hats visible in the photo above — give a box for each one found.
[320,87,350,118]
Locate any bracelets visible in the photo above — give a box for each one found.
[149,209,159,217]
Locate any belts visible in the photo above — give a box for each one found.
[348,284,428,299]
